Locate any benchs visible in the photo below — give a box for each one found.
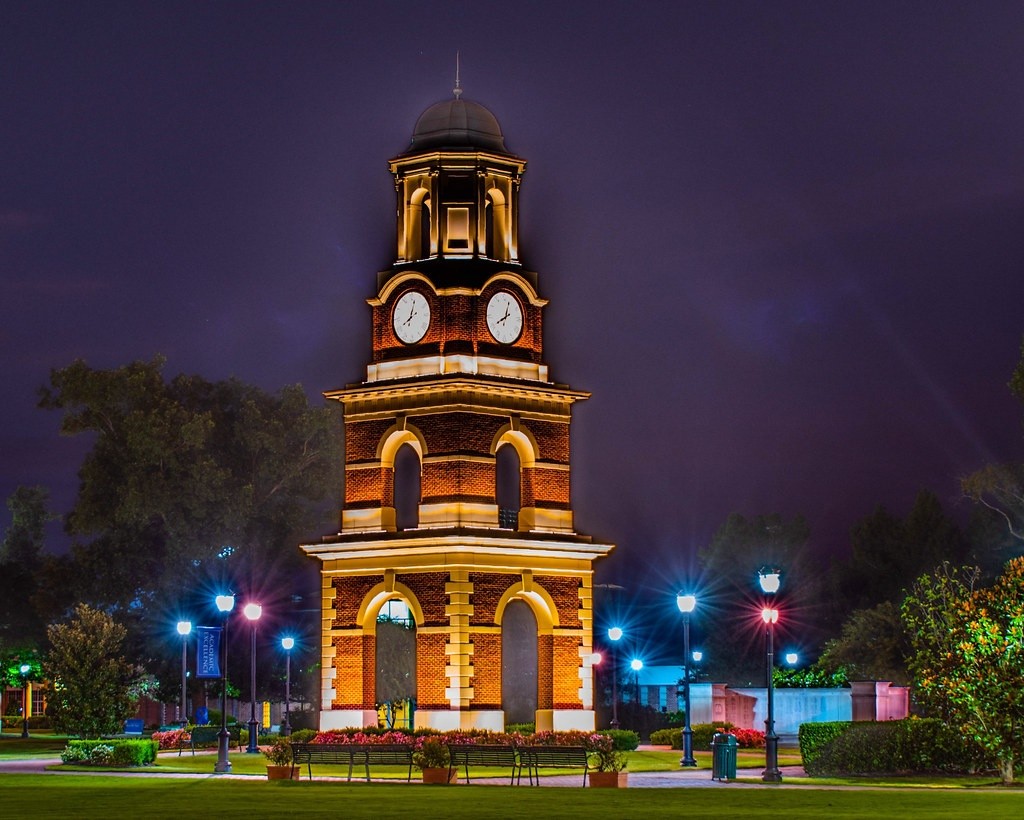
[290,743,420,783]
[447,743,604,788]
[179,725,242,756]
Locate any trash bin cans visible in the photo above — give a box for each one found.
[710,733,740,783]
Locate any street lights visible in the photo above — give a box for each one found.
[630,658,643,702]
[607,625,623,728]
[282,634,295,737]
[215,592,236,773]
[691,651,703,682]
[177,618,192,727]
[243,599,262,753]
[677,587,698,767]
[757,564,784,783]
[20,664,30,738]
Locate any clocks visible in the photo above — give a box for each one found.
[394,292,430,344]
[486,292,522,343]
